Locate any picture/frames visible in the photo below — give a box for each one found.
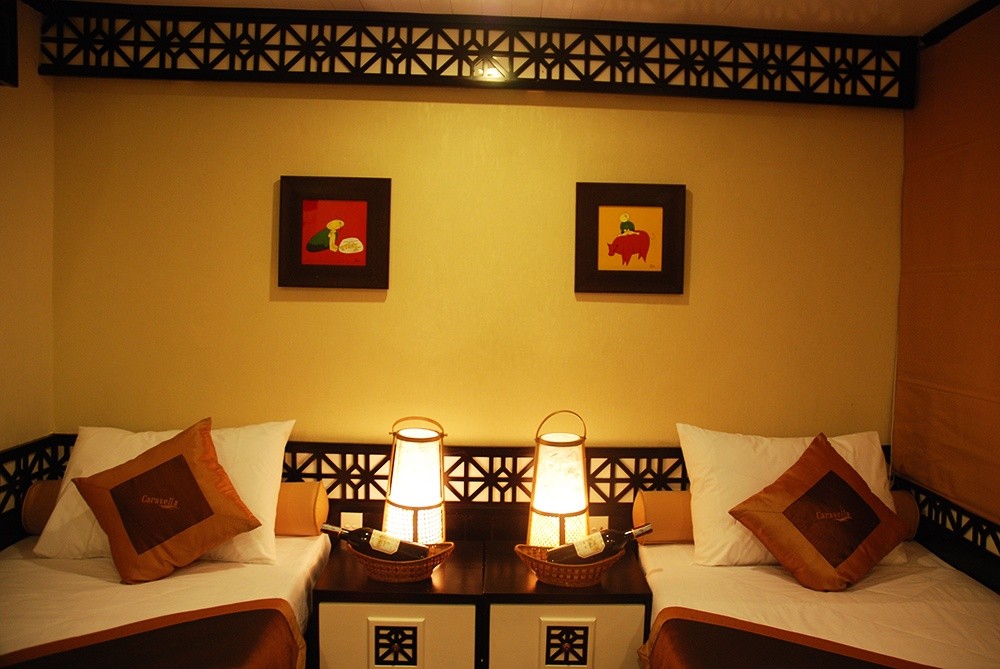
[278,174,390,291]
[574,182,686,295]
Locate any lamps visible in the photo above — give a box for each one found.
[379,416,447,547]
[527,411,589,550]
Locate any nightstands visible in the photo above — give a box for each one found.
[311,536,481,669]
[484,540,652,669]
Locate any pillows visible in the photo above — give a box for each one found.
[633,490,921,546]
[730,432,909,593]
[676,422,906,565]
[33,420,294,565]
[24,481,328,536]
[72,418,261,585]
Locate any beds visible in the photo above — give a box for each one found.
[639,447,1000,669]
[0,432,330,669]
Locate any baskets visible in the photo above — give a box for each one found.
[515,544,625,586]
[347,543,455,582]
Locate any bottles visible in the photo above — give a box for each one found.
[546,523,653,564]
[319,522,429,562]
